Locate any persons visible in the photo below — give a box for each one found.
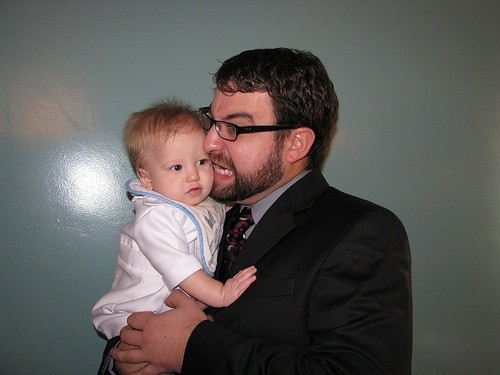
[100,46,414,375]
[90,93,259,375]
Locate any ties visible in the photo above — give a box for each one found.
[222,209,254,272]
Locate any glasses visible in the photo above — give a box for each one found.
[198,106,296,141]
